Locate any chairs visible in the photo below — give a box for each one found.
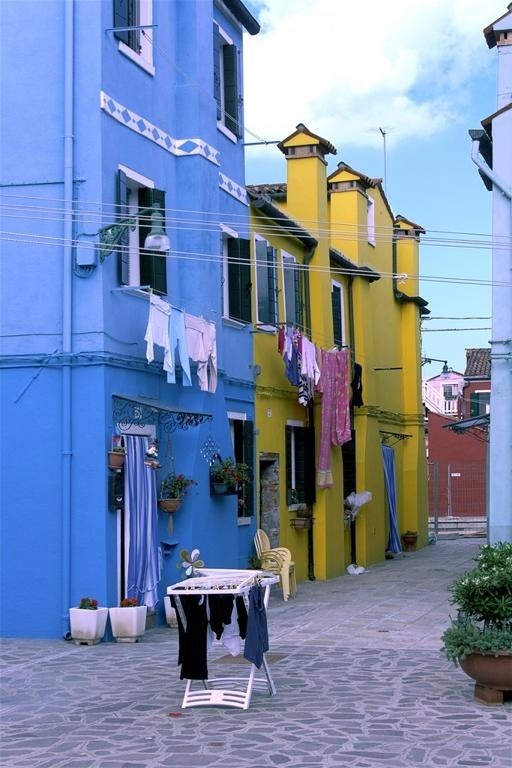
[254,529,297,601]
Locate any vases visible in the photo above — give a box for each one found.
[162,597,179,628]
[69,606,109,645]
[457,642,512,705]
[110,606,148,643]
[157,499,181,512]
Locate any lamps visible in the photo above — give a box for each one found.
[100,202,171,264]
[420,357,452,379]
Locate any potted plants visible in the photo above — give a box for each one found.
[210,456,252,494]
[294,505,313,528]
[401,529,417,543]
[109,446,127,467]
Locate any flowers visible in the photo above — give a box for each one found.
[439,538,512,667]
[79,597,98,610]
[160,473,193,499]
[120,597,137,607]
